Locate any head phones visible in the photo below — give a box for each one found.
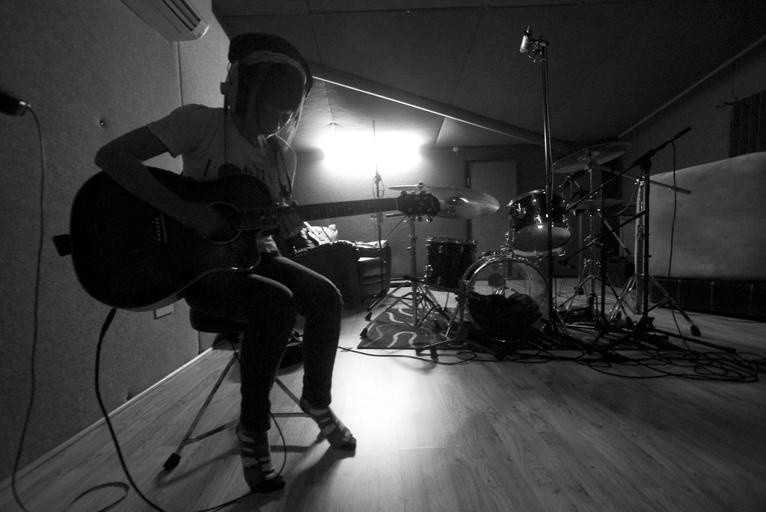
[220,46,313,113]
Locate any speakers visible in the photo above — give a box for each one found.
[709,277,766,325]
[650,275,709,314]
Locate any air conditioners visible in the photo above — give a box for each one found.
[123,0,210,42]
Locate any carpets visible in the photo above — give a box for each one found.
[356,307,680,350]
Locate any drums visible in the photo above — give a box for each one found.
[508,188,572,252]
[425,236,476,293]
[461,253,549,343]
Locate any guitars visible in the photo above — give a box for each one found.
[69,165,441,312]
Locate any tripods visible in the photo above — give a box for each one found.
[596,162,703,338]
[558,217,633,332]
[364,219,412,310]
[360,217,459,342]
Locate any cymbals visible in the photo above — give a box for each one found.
[388,185,500,221]
[550,139,631,174]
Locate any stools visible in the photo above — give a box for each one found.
[164,309,323,471]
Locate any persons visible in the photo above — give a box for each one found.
[94,30,360,495]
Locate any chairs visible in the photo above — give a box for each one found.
[295,221,391,310]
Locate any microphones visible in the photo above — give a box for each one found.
[517,25,533,53]
